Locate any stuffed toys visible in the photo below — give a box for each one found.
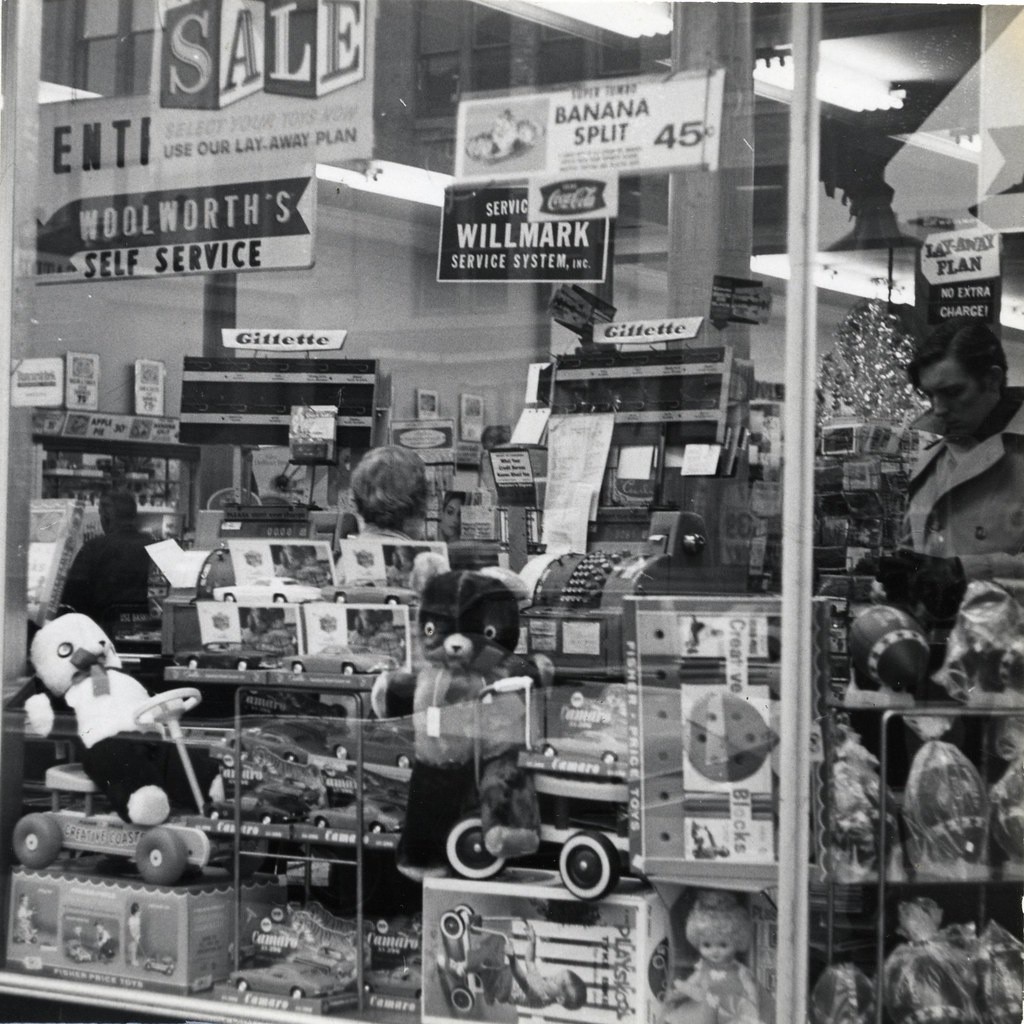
[18,611,225,827]
[367,553,547,866]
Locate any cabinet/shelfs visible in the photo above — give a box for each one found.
[824,700,1024,1024]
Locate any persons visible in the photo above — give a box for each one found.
[128,903,141,968]
[330,444,431,593]
[56,487,156,641]
[15,893,33,947]
[94,924,117,962]
[659,894,760,1024]
[447,913,585,1016]
[439,493,465,539]
[876,315,1024,600]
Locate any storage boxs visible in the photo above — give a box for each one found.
[421,867,676,1023]
[621,595,832,876]
[7,864,289,995]
